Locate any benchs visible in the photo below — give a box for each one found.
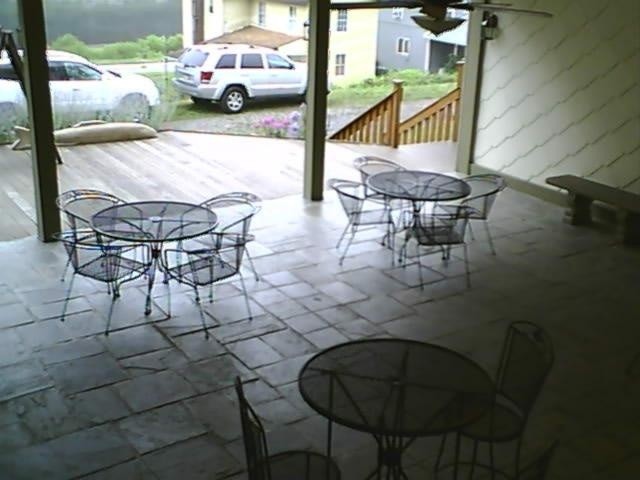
[546,175,640,246]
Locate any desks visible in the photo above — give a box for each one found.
[90,201,220,314]
[365,171,473,262]
[299,337,496,479]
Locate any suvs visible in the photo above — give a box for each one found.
[0,47,161,133]
[171,42,332,114]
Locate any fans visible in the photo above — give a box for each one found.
[330,0,552,35]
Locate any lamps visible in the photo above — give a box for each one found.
[481,15,498,39]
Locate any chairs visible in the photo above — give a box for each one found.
[52,232,152,336]
[399,201,480,291]
[435,320,557,480]
[234,374,341,479]
[180,192,262,302]
[56,189,151,295]
[327,178,392,266]
[354,156,418,249]
[432,175,508,258]
[164,232,254,339]
